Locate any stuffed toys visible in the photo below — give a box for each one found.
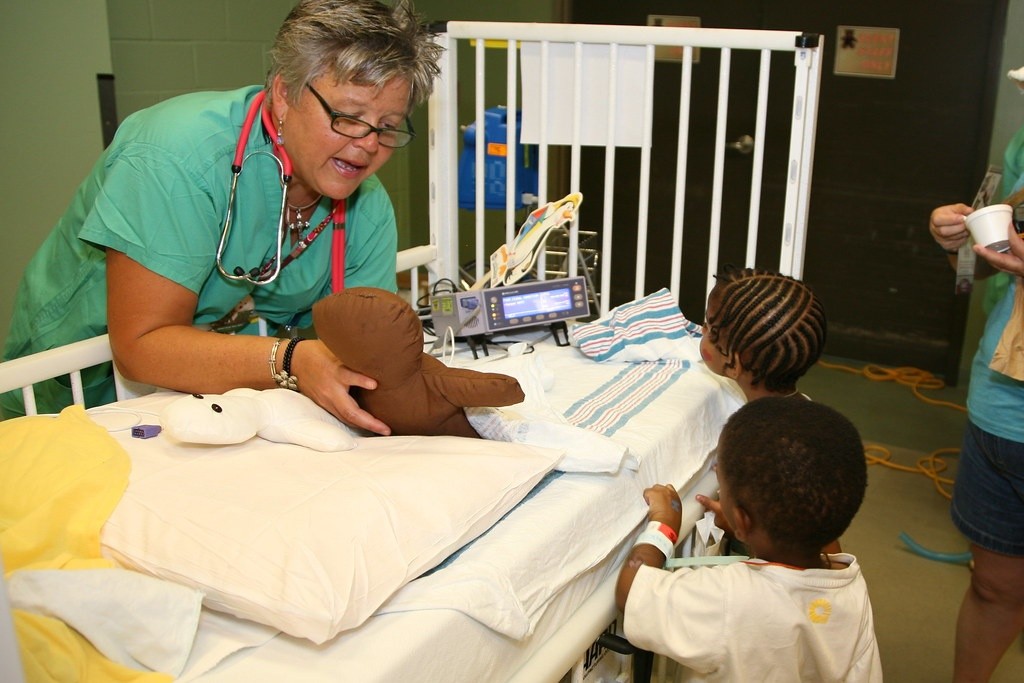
[128,383,378,457]
[304,286,527,445]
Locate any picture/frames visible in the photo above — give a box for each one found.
[833,25,899,80]
[647,14,702,64]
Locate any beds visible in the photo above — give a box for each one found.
[1,312,749,683]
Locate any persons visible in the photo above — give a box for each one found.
[612,388,887,683]
[694,269,849,564]
[1,0,451,431]
[930,55,1024,683]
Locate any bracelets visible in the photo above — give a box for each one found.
[631,530,674,564]
[642,520,678,544]
[267,335,287,383]
[272,336,310,394]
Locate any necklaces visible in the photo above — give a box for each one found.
[781,388,802,397]
[264,133,326,248]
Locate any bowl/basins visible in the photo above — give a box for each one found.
[965,203,1013,253]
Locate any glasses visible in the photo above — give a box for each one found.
[302,80,416,148]
